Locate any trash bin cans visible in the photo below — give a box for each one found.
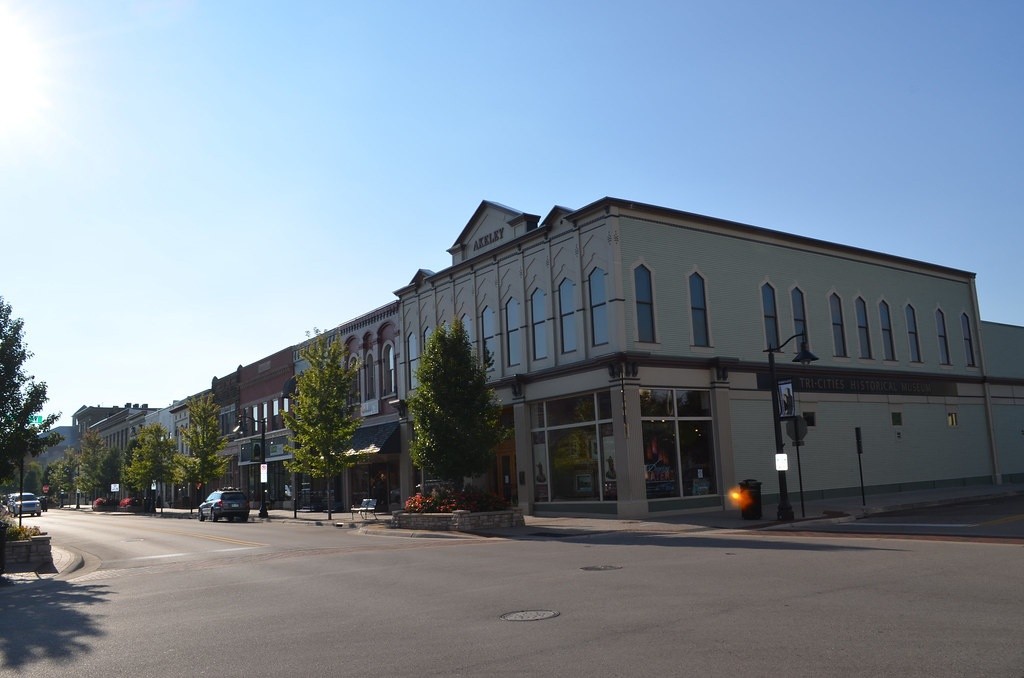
[737,478,763,520]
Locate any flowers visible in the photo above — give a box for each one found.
[94,497,112,506]
[403,480,511,514]
[120,496,137,507]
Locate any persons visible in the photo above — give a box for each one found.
[43,497,47,512]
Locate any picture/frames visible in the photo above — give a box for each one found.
[573,470,595,497]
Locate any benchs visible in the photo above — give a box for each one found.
[350,499,378,520]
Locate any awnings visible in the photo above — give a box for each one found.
[342,421,401,455]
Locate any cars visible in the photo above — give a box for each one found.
[198,487,250,522]
[8,493,41,517]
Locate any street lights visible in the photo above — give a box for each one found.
[762,329,820,522]
[232,415,268,518]
